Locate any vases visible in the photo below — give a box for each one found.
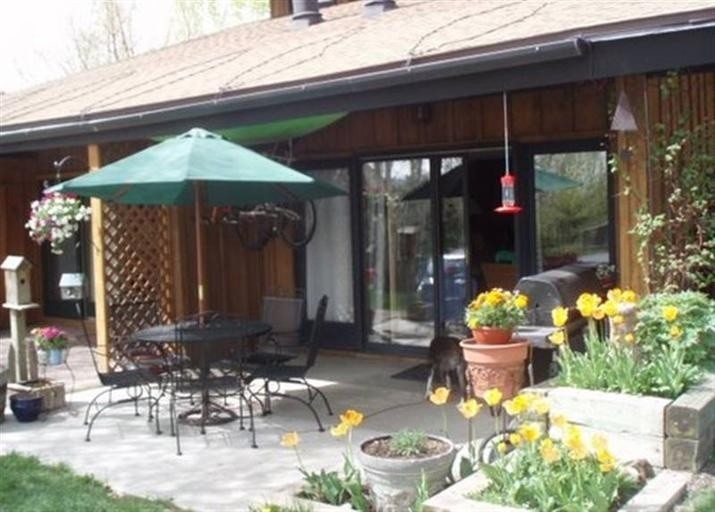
[7,393,44,423]
[36,347,62,366]
[459,325,529,401]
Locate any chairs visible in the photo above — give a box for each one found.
[110,299,196,407]
[261,296,304,345]
[75,302,169,442]
[239,296,333,434]
[167,310,258,456]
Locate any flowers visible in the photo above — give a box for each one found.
[462,286,531,329]
[29,326,77,364]
[21,184,90,262]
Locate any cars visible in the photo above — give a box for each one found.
[416,249,466,302]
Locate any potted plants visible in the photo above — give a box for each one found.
[355,428,457,512]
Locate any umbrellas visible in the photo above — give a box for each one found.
[43,126,352,328]
[401,164,584,199]
